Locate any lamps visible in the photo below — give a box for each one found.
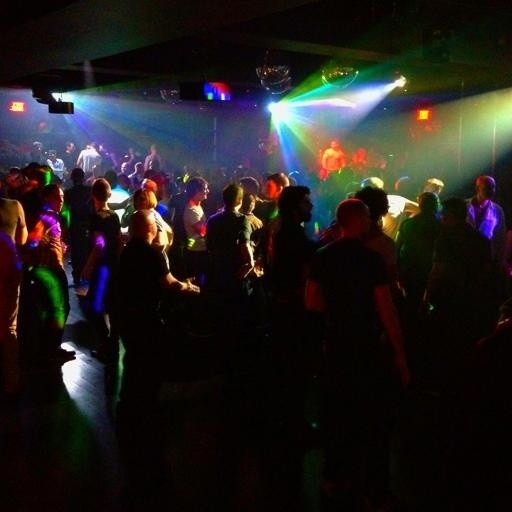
[256,46,359,95]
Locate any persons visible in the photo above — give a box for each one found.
[2,139,510,506]
